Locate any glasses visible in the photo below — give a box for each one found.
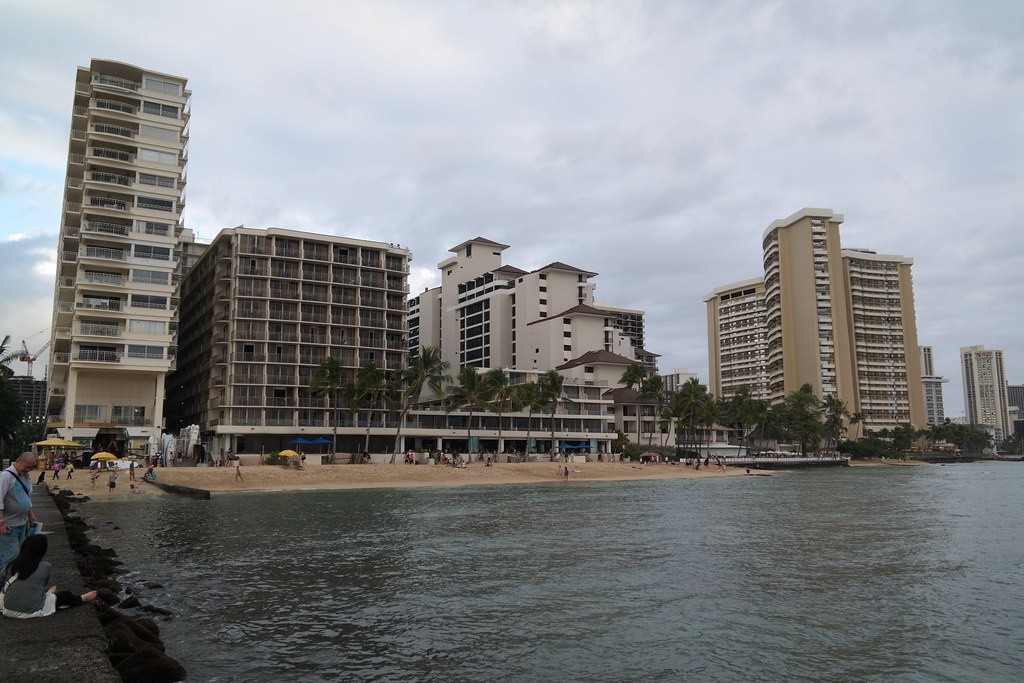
[21,460,34,470]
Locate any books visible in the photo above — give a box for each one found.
[25,522,43,538]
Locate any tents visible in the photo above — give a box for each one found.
[553,443,595,449]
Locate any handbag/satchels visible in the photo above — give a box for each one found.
[302,456,306,460]
[0,590,6,610]
[71,469,73,472]
[406,454,409,458]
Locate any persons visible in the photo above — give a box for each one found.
[362,449,370,464]
[405,445,521,468]
[2,534,98,619]
[260,445,266,464]
[0,452,37,590]
[49,447,242,493]
[299,451,307,466]
[561,448,720,480]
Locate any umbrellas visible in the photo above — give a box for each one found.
[91,452,117,469]
[278,450,300,469]
[288,437,332,454]
[29,438,82,455]
